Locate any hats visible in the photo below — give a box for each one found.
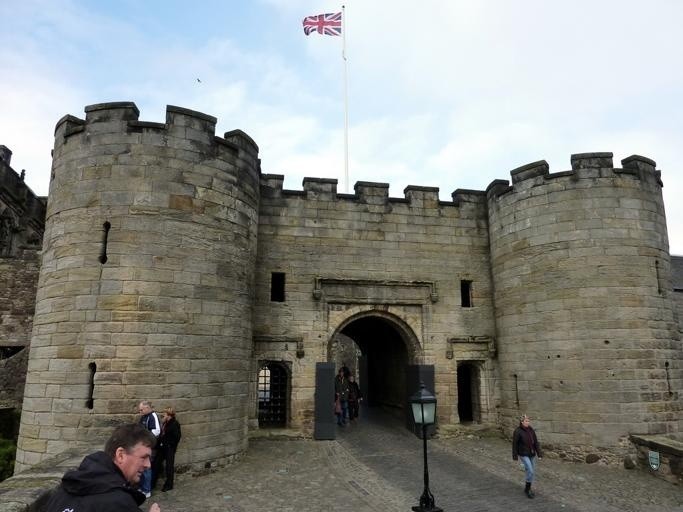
[520,414,531,422]
[163,406,175,414]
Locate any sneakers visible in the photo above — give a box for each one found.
[138,483,173,498]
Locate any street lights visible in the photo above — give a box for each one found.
[411,381,443,511]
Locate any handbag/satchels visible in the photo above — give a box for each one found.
[335,394,342,414]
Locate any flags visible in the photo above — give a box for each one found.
[302,11,341,38]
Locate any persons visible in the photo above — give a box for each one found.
[34,420,160,512]
[511,414,543,499]
[156,406,181,492]
[345,374,364,424]
[332,368,349,426]
[133,399,161,499]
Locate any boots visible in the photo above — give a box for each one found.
[525,482,534,498]
